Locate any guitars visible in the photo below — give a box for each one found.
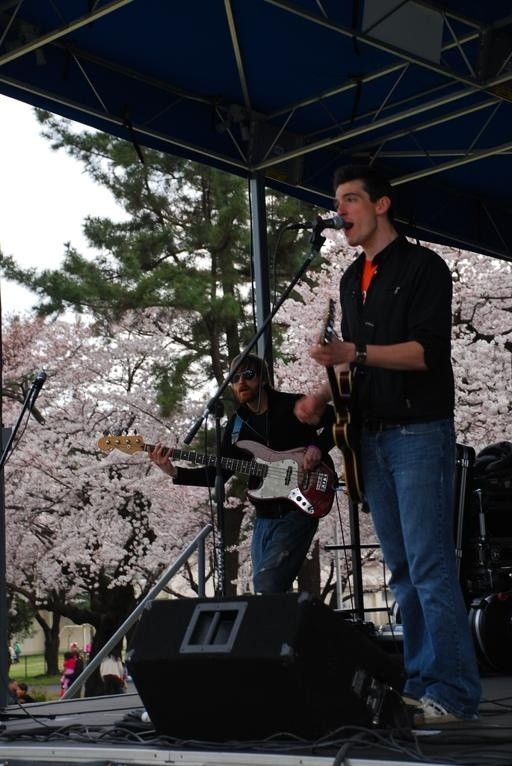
[320,299,367,503]
[96,427,338,519]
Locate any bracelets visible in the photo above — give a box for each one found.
[350,342,367,363]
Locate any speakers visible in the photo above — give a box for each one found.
[127,595,415,746]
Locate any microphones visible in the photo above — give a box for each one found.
[30,371,47,408]
[290,215,345,230]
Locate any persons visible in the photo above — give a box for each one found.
[6,638,135,704]
[294,164,483,726]
[144,354,341,598]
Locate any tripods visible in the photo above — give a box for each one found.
[0,386,56,720]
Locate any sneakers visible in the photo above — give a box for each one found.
[400,695,465,724]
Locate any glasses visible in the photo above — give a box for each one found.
[230,368,257,383]
